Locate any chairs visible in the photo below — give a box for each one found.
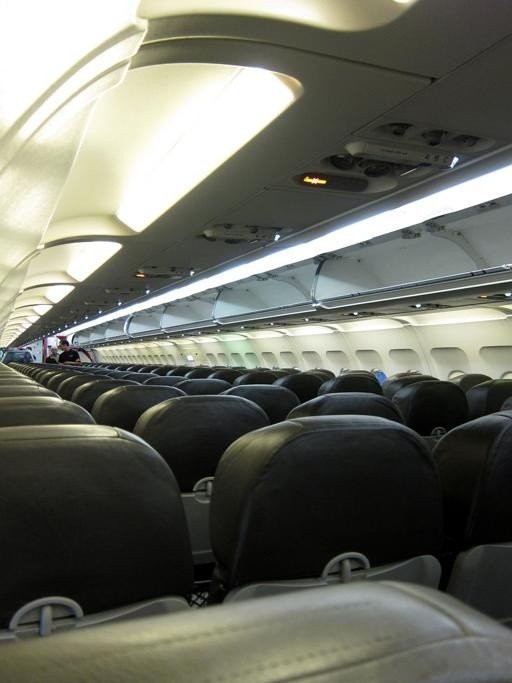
[392,379,470,452]
[133,395,274,593]
[1,422,193,646]
[1,579,512,681]
[209,415,443,607]
[431,408,511,626]
[1,357,512,427]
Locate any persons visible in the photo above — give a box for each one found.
[46,346,60,364]
[26,347,37,364]
[50,339,80,365]
